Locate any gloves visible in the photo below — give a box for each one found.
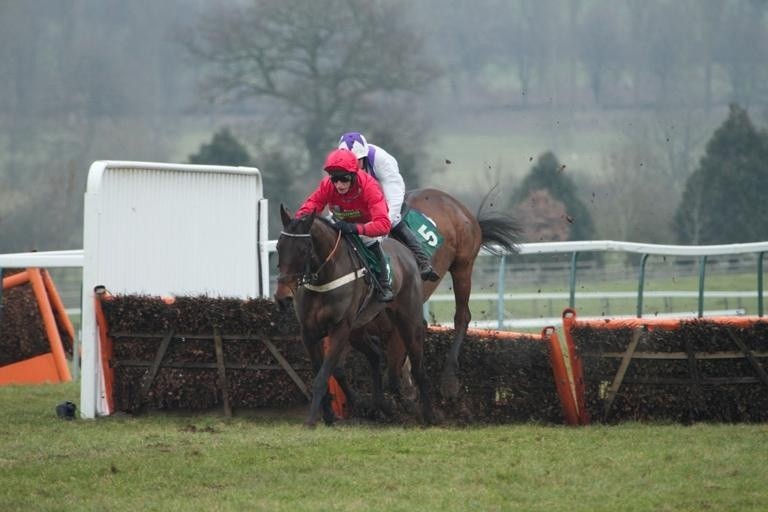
[336,220,359,235]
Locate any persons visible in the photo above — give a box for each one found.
[293,149,394,302]
[338,132,441,283]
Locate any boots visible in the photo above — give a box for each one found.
[368,240,392,301]
[391,221,441,282]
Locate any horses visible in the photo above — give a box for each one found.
[332,181,525,425]
[273,203,445,433]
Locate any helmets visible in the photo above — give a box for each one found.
[323,148,359,173]
[338,132,369,160]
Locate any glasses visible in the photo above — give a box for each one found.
[330,173,352,183]
